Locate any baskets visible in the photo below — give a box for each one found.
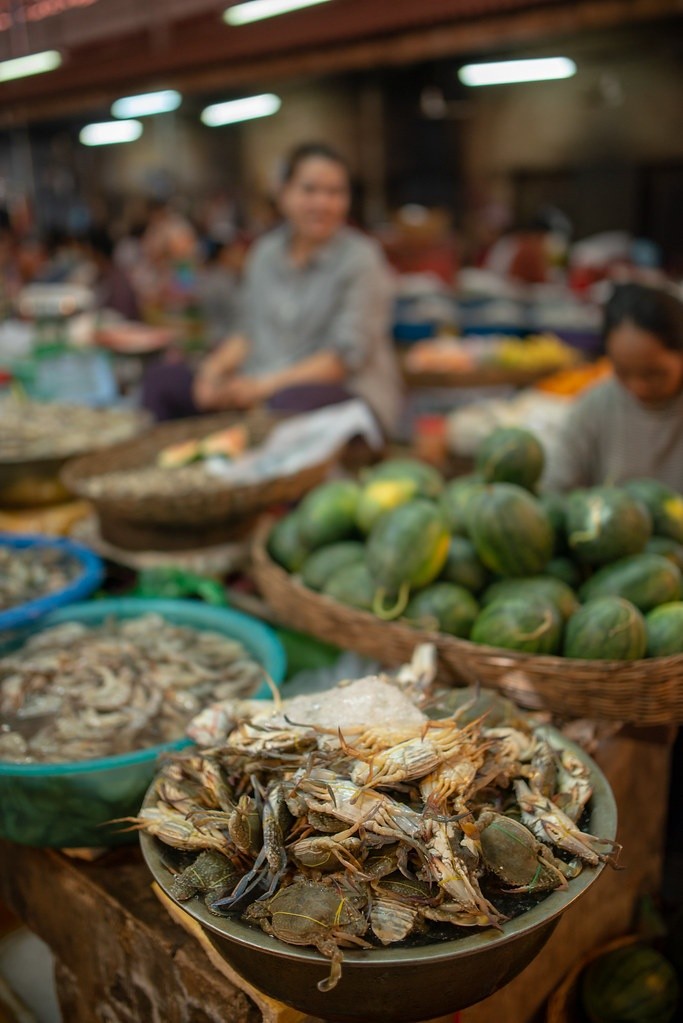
[60,401,360,518]
[258,434,683,727]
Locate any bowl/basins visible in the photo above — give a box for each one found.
[0,597,287,844]
[0,531,108,634]
[135,706,621,1023]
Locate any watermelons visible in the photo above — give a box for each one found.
[581,946,677,1022]
[265,424,682,663]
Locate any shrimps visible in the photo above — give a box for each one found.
[110,614,262,679]
[0,615,109,847]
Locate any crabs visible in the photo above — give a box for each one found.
[110,678,626,992]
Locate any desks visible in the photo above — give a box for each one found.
[0,437,683,1023]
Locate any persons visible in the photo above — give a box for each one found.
[537,281,682,502]
[187,142,407,445]
